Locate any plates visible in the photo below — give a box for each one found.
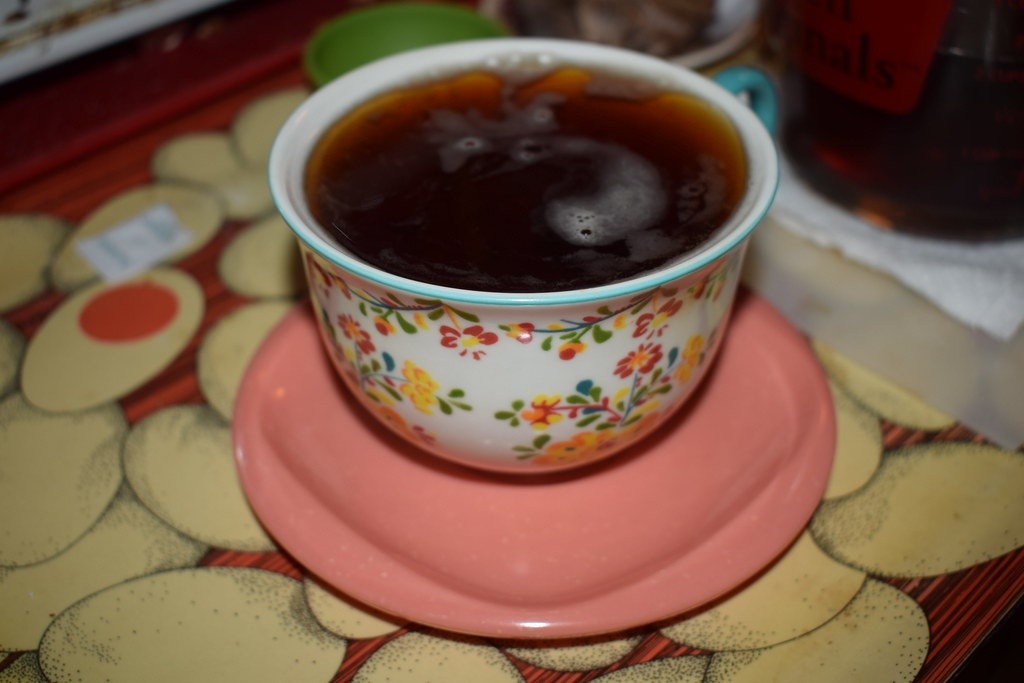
[233,281,837,643]
[479,0,761,71]
[304,2,508,88]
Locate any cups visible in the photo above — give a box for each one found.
[269,38,781,474]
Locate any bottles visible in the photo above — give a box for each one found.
[755,0,1024,243]
